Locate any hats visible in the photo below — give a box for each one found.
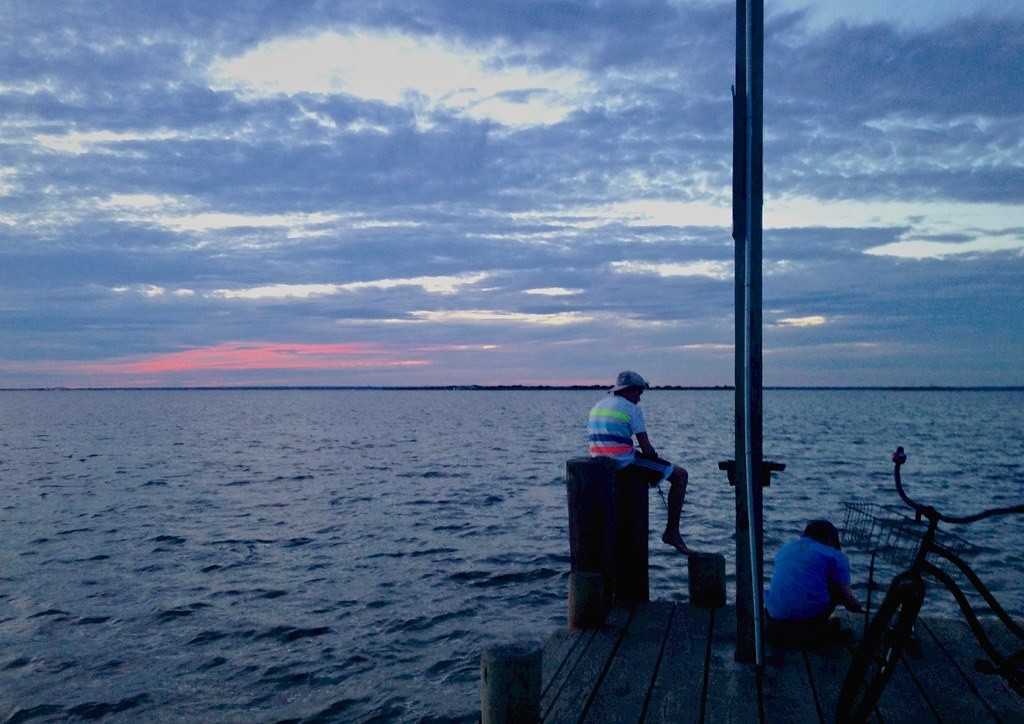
[605,370,645,393]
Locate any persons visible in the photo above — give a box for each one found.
[588,371,692,555]
[763,519,862,638]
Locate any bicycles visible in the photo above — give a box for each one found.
[829,444,1024,720]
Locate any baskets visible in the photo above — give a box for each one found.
[840,501,980,589]
